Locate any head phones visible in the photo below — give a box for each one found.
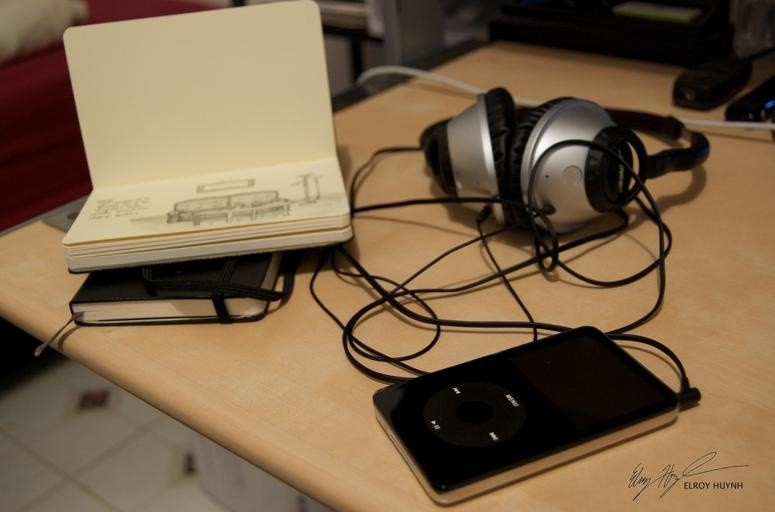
[422,89,710,232]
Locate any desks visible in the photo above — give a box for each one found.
[0,40,775,512]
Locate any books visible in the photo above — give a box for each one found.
[34,1,355,358]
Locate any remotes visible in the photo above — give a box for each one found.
[724,71,774,123]
